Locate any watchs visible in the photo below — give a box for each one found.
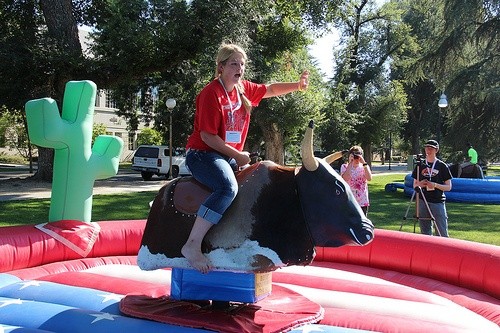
[434,183,437,187]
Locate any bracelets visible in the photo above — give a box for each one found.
[363,163,367,168]
[298,83,303,91]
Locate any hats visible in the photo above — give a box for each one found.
[466,143,472,149]
[424,140,439,150]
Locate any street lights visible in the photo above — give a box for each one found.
[437,87,449,145]
[165,98,177,179]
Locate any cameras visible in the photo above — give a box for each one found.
[353,151,361,160]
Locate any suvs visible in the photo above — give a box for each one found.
[130,144,192,181]
[314,150,346,172]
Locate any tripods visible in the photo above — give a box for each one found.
[399,160,442,237]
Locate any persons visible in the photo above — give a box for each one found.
[411,140,453,238]
[458,143,478,178]
[181,43,310,274]
[340,146,372,218]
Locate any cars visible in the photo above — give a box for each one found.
[392,153,489,175]
